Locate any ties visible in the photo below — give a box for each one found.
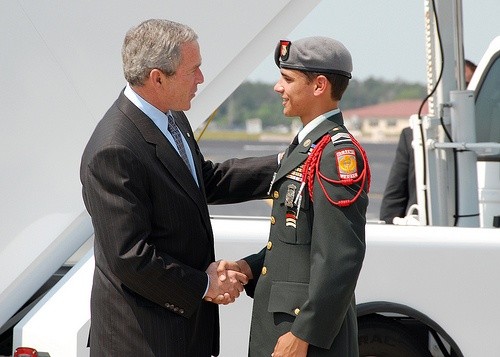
[287,134,299,159]
[167,116,193,176]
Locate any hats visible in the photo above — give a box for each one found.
[274,37,352,79]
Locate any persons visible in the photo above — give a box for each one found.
[380,60,477,225]
[204,36,371,357]
[80,17,285,357]
